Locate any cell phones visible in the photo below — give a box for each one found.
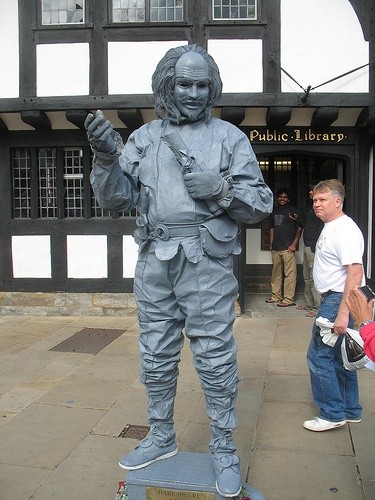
[358,285,375,301]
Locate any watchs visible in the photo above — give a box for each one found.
[359,320,374,329]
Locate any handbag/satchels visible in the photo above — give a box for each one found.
[314,315,372,371]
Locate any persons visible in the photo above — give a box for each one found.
[265,188,304,307]
[346,285,375,363]
[303,179,365,431]
[296,182,324,317]
[83,44,274,498]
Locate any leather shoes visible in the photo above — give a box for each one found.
[295,304,319,316]
[265,298,295,307]
[302,416,362,432]
